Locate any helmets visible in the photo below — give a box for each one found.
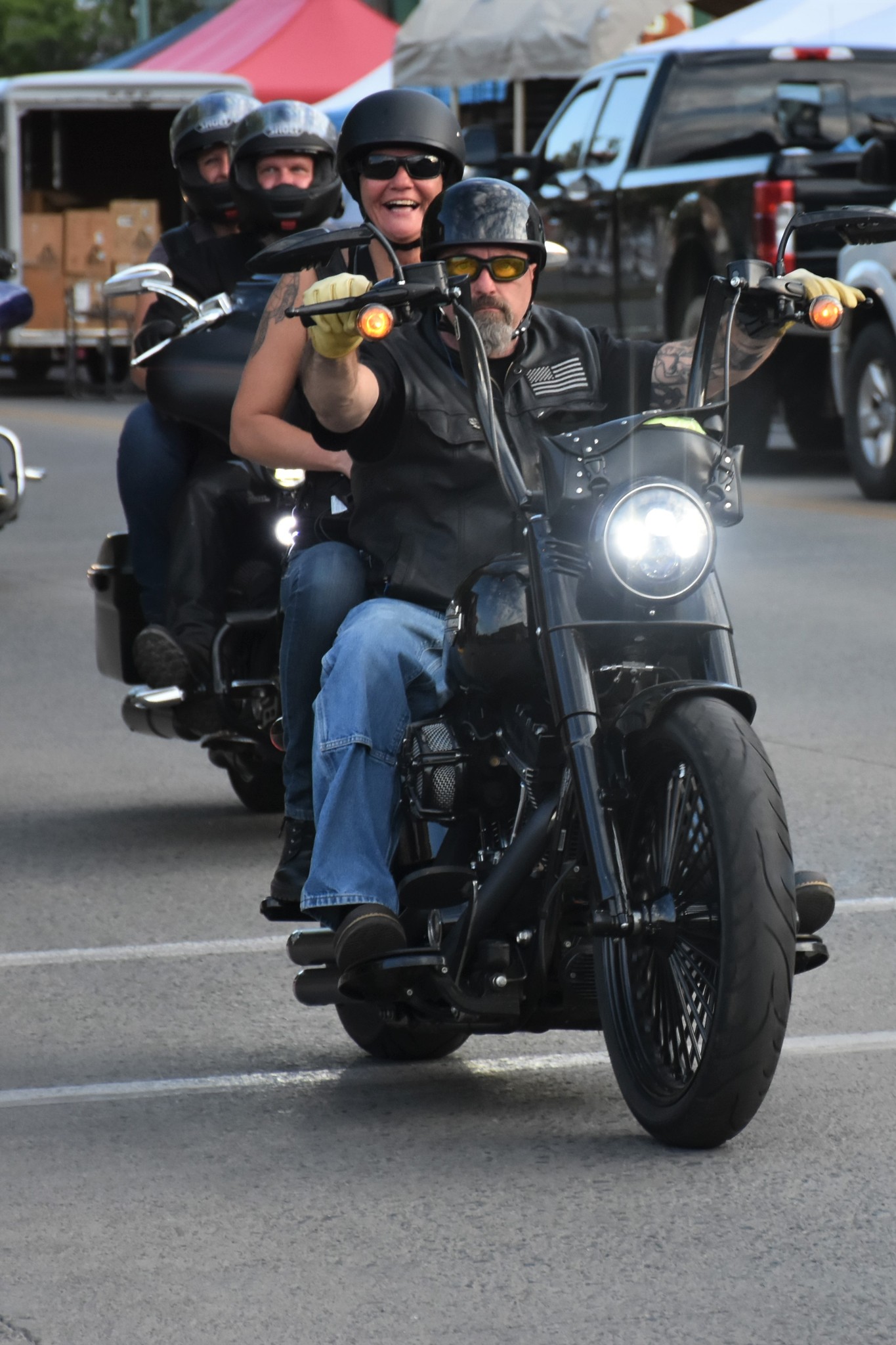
[169,90,263,227]
[334,89,468,188]
[233,100,346,235]
[420,176,549,274]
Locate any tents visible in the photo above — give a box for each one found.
[126,1,509,197]
[84,8,222,73]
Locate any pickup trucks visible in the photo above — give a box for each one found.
[457,45,895,463]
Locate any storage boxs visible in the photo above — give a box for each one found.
[23,183,160,338]
[86,531,148,688]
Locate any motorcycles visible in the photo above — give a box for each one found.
[87,264,348,820]
[249,202,896,1146]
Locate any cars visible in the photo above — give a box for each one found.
[827,204,895,499]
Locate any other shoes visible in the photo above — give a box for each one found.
[132,625,224,740]
[795,872,833,934]
[333,904,407,972]
[270,813,314,901]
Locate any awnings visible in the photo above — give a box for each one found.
[390,0,693,156]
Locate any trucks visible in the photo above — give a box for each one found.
[0,69,258,381]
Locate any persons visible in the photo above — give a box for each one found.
[220,88,470,922]
[98,74,266,743]
[131,100,343,736]
[298,174,867,979]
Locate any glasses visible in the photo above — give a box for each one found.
[444,252,531,282]
[356,152,443,180]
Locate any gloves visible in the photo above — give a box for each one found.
[783,267,867,309]
[300,272,370,360]
